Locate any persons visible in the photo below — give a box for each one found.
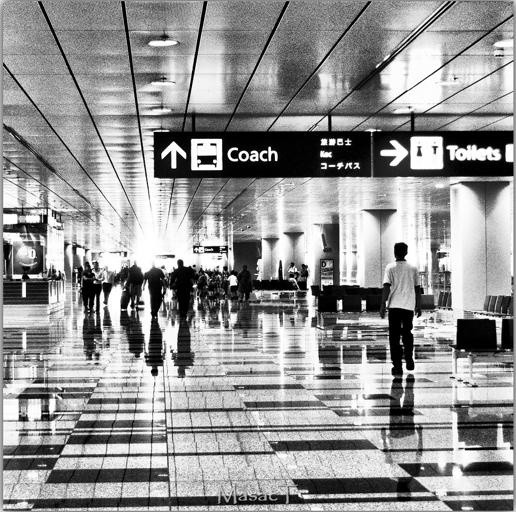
[43,265,64,280]
[76,259,251,315]
[381,375,423,501]
[380,243,422,375]
[288,262,309,290]
[83,306,252,378]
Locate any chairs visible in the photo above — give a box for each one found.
[251,280,514,387]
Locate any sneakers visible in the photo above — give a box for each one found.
[405,356,414,370]
[392,367,403,375]
